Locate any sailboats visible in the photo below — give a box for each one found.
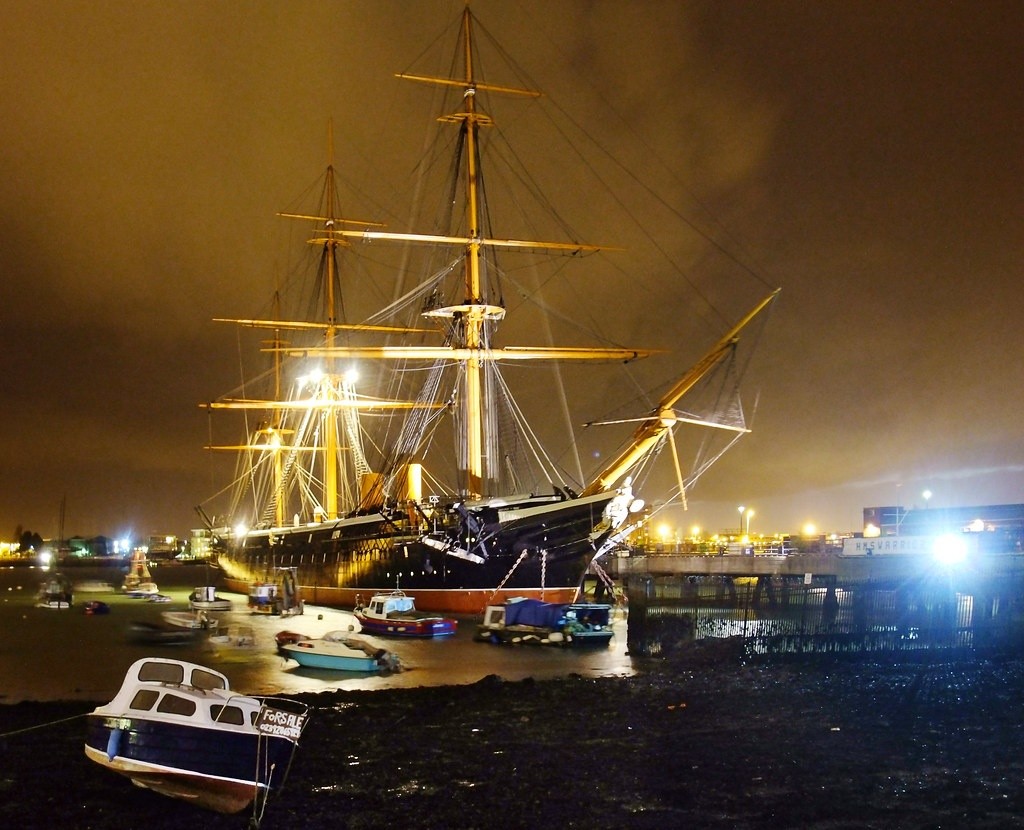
[193,0,782,621]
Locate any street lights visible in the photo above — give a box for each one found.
[896,489,932,536]
[746,508,755,533]
[738,506,744,533]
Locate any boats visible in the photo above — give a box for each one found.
[248,566,303,616]
[80,601,111,615]
[160,610,219,630]
[275,631,399,671]
[34,592,73,609]
[476,599,614,646]
[82,657,308,815]
[352,574,458,638]
[188,585,232,612]
